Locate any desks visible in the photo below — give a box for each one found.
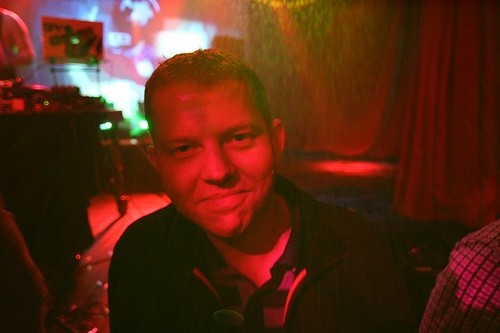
[0,111,122,196]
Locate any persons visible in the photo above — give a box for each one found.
[0,9,418,333]
[419,218,500,333]
